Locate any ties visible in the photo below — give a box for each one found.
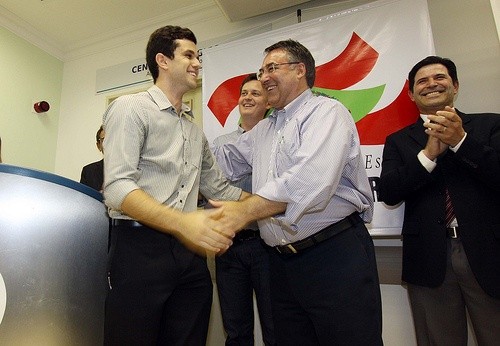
[430,117,456,228]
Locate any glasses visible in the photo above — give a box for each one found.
[257,61,299,81]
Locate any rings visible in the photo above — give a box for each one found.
[442,127,447,133]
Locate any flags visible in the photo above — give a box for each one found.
[202,0,436,235]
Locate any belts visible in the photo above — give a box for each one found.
[270,211,362,255]
[112,218,146,227]
[447,226,460,239]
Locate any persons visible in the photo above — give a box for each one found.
[378,56,500,346]
[80,124,105,194]
[210,39,381,346]
[206,76,281,346]
[103,27,254,346]
